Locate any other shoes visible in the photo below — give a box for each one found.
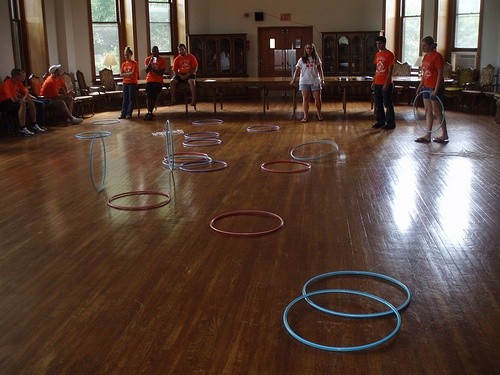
[67,118,71,123]
[126,115,131,119]
[381,124,395,129]
[71,117,83,123]
[372,122,385,127]
[144,113,153,120]
[169,101,176,105]
[118,116,126,119]
[191,102,196,106]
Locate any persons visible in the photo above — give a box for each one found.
[213,50,231,73]
[170,44,199,106]
[118,46,139,119]
[39,64,83,124]
[0,68,47,137]
[372,36,396,130]
[415,36,450,143]
[144,46,167,120]
[290,42,326,123]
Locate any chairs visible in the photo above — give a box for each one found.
[390,56,500,117]
[0,67,172,138]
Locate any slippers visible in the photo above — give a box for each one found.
[316,114,324,121]
[433,138,449,143]
[415,138,431,142]
[300,118,310,122]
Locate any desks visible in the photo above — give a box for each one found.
[116,76,452,114]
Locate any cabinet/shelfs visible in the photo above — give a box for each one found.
[188,33,249,100]
[322,30,381,98]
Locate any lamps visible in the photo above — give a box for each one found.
[103,55,117,70]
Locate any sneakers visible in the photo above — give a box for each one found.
[17,127,34,137]
[29,124,47,133]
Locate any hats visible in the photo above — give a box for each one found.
[48,64,61,74]
